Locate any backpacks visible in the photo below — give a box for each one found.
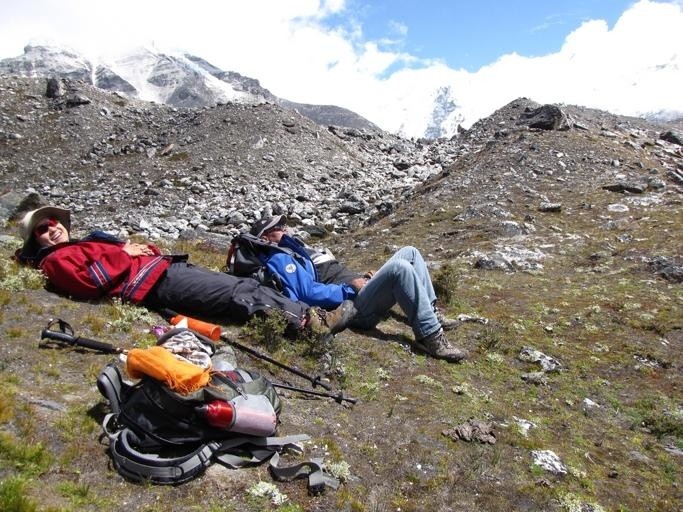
[95,327,284,486]
[221,232,307,294]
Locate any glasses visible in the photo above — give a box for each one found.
[267,224,287,232]
[36,218,59,235]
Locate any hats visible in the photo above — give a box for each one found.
[249,215,287,239]
[15,206,71,262]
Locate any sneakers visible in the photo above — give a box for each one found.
[424,306,458,330]
[305,299,358,346]
[412,327,466,363]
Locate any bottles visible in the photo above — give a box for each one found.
[169,315,222,342]
[193,398,278,438]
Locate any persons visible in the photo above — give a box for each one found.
[19,207,358,342]
[249,213,466,362]
